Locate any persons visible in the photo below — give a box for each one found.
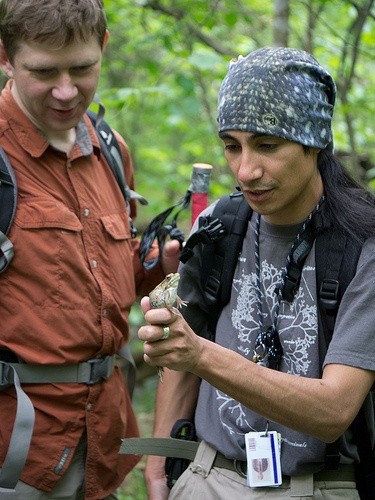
[1,0,186,500]
[137,45,375,500]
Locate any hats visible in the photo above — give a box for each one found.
[216,45,336,153]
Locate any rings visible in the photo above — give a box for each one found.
[163,327,171,339]
